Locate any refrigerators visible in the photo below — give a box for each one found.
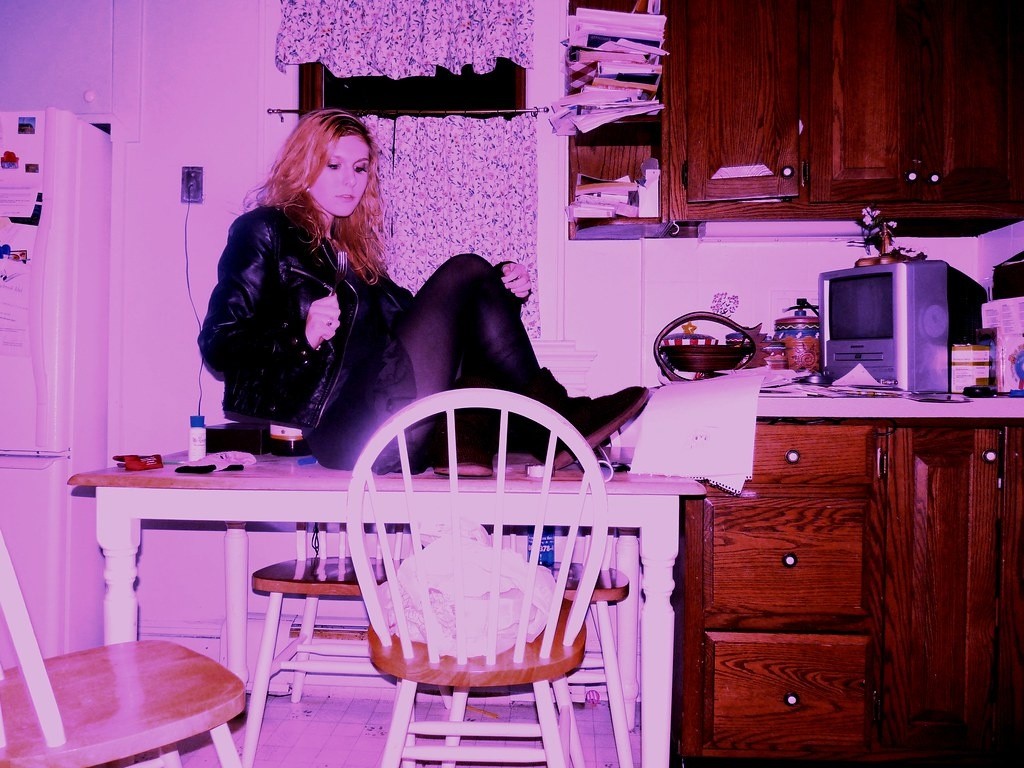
[1,109,111,675]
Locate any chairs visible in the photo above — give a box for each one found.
[492,526,633,768]
[0,532,246,768]
[244,522,416,768]
[347,387,608,768]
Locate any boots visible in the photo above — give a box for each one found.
[526,368,649,470]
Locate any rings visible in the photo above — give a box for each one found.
[326,318,333,326]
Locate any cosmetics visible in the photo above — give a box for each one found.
[188,416,207,461]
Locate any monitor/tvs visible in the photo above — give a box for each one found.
[817,259,988,394]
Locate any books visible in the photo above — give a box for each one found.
[549,7,670,138]
[572,173,640,219]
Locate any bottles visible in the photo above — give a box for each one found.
[188,415,205,462]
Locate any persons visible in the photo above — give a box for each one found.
[195,108,649,477]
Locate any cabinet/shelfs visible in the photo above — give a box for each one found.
[685,0,799,203]
[808,0,1024,205]
[881,427,1024,767]
[682,417,877,761]
[568,0,670,240]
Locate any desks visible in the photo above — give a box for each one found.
[68,450,706,768]
